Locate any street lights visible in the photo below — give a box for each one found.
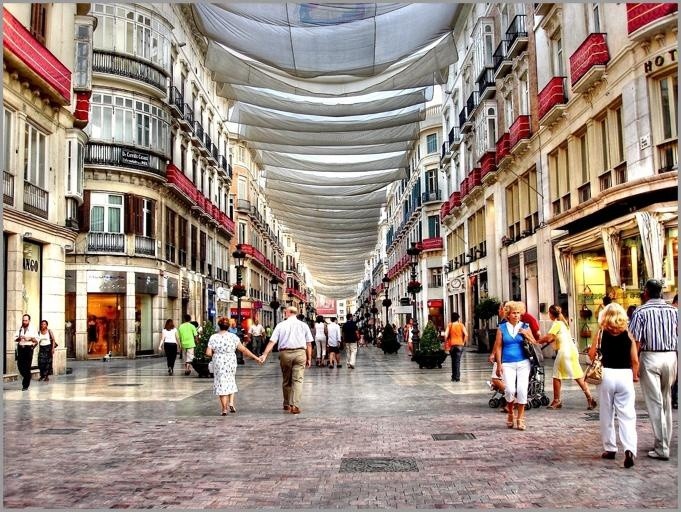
[299,299,304,316]
[269,275,280,353]
[382,272,393,325]
[371,288,379,344]
[364,298,373,343]
[305,306,317,326]
[405,241,422,361]
[352,304,364,334]
[231,243,247,364]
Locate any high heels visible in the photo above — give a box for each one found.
[623,449,635,469]
[586,398,597,410]
[546,398,562,410]
[601,451,616,460]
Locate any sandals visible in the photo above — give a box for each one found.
[506,412,515,428]
[516,418,527,430]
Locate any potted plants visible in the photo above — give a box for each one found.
[474,298,500,352]
[190,318,217,378]
[412,318,449,369]
[378,323,403,355]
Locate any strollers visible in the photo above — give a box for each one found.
[486,341,551,411]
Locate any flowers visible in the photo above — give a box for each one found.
[405,280,424,295]
[228,284,247,297]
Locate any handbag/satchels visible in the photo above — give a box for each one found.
[579,304,593,319]
[582,284,593,300]
[443,337,452,355]
[583,328,603,385]
[49,330,58,348]
[522,322,544,365]
[580,323,591,338]
[207,361,215,374]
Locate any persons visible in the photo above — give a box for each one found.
[537,306,596,410]
[487,301,517,412]
[519,301,539,342]
[496,301,536,430]
[588,280,678,409]
[178,315,199,375]
[259,306,314,413]
[159,319,180,376]
[87,316,98,354]
[229,314,414,368]
[37,320,55,381]
[206,318,261,415]
[628,280,678,460]
[444,313,468,381]
[14,314,40,391]
[589,303,640,468]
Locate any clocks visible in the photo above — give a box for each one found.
[451,279,462,290]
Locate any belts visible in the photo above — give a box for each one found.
[280,348,304,351]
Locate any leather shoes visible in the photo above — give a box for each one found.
[647,449,670,461]
[218,403,237,417]
[282,402,302,414]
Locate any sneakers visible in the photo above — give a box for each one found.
[167,367,174,376]
[347,363,355,369]
[328,363,343,369]
